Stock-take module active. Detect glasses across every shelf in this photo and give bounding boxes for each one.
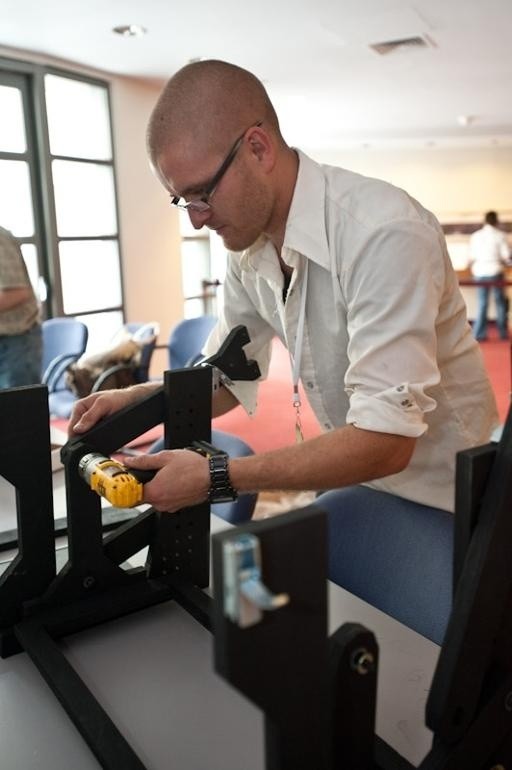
[170,123,263,211]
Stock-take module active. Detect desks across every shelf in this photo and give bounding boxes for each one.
[0,421,454,769]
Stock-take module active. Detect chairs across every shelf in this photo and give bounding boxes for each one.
[315,486,454,649]
[49,323,157,419]
[147,428,261,525]
[38,319,86,391]
[136,311,224,379]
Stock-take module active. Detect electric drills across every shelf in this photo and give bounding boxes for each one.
[77,440,228,509]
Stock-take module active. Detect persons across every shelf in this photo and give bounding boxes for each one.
[465,211,512,342]
[0,225,43,389]
[67,60,499,514]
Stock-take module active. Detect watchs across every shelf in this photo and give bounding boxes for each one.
[206,449,238,504]
